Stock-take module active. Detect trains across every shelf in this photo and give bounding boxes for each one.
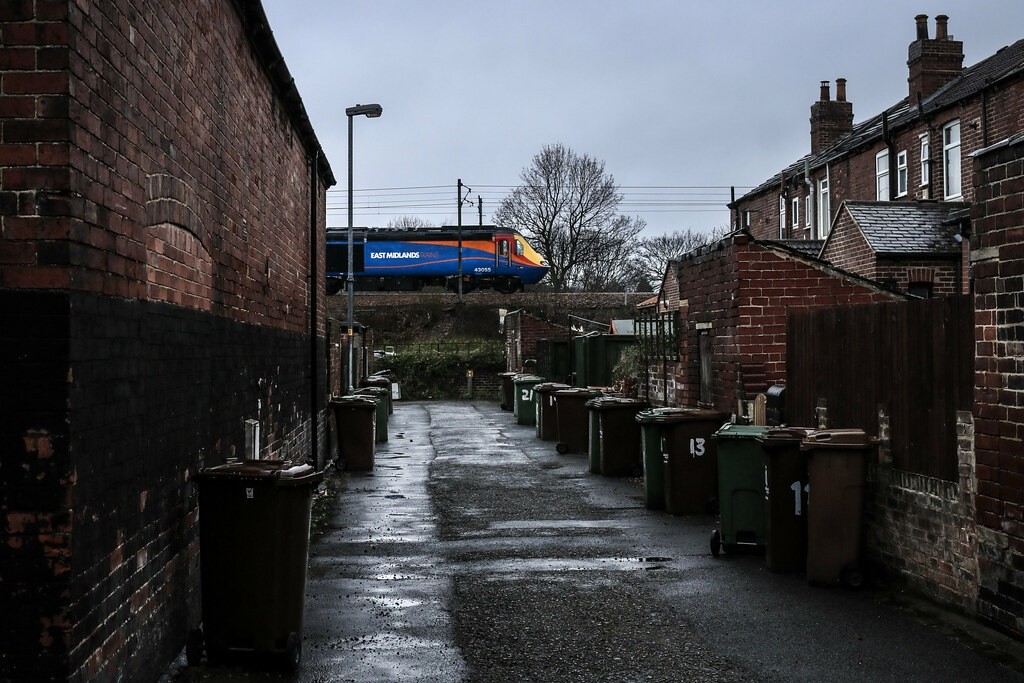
[325,224,551,297]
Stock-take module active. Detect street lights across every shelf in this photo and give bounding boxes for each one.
[346,102,383,393]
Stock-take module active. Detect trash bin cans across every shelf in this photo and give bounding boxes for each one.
[512,373,546,425]
[555,388,603,454]
[360,369,393,414]
[634,406,724,516]
[708,423,804,571]
[332,396,376,473]
[584,397,641,477]
[353,386,388,444]
[499,371,517,411]
[184,460,324,671]
[532,382,571,441]
[802,430,880,599]
